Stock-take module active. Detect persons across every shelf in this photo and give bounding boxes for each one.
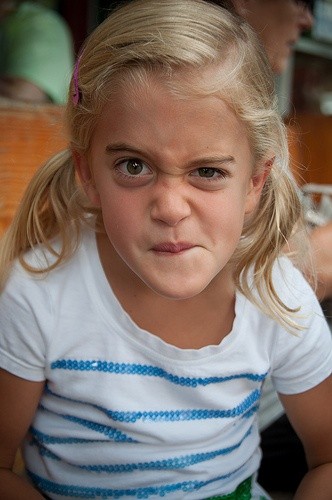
[2,0,76,106]
[2,0,332,498]
